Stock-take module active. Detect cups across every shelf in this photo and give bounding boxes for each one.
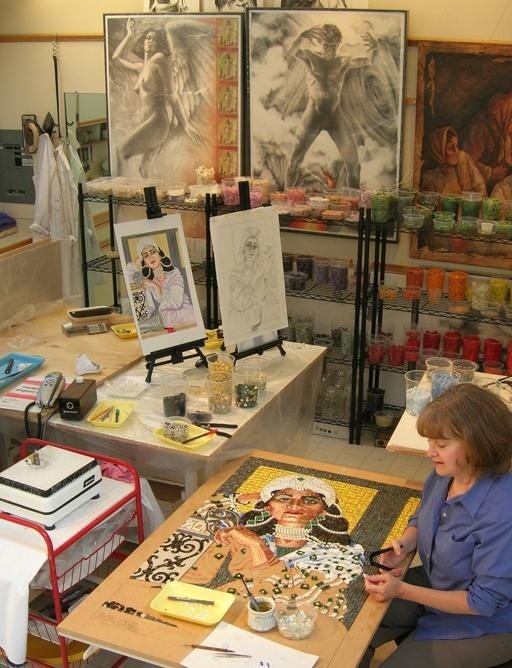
[246,596,279,633]
[111,306,121,323]
[272,603,318,640]
[159,350,269,421]
[87,172,511,238]
[281,255,512,427]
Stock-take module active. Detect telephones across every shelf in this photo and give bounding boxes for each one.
[34,371,65,408]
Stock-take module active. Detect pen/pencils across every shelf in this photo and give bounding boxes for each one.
[168,596,214,606]
[5,359,13,374]
[182,429,217,444]
[90,406,119,423]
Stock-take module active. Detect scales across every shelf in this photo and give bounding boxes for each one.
[0,444,103,530]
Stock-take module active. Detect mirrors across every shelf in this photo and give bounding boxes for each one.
[63,91,115,307]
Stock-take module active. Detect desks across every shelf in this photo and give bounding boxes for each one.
[0,302,143,455]
[386,364,512,456]
[44,325,329,547]
[57,444,425,667]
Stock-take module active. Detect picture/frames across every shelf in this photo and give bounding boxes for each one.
[103,9,244,189]
[245,7,407,244]
[409,37,512,276]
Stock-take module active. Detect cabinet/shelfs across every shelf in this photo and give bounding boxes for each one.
[78,184,222,329]
[356,204,512,448]
[0,434,148,668]
[211,196,386,447]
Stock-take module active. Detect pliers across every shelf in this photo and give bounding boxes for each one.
[192,421,238,438]
[364,545,403,571]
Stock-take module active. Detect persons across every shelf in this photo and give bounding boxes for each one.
[419,89,512,203]
[111,19,207,180]
[362,384,512,668]
[210,474,366,606]
[134,237,196,332]
[278,22,381,189]
[224,224,281,330]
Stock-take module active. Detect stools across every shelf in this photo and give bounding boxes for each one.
[391,631,512,667]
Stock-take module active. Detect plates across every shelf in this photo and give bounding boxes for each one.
[113,322,140,339]
[154,420,216,450]
[149,578,235,626]
[108,374,150,398]
[85,399,136,428]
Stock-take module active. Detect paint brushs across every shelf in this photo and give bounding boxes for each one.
[191,644,251,658]
